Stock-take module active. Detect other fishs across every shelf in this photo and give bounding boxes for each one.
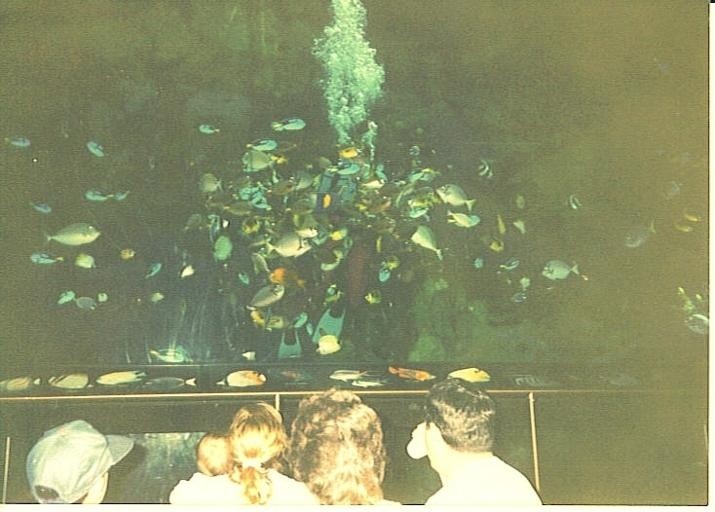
[145,116,483,365]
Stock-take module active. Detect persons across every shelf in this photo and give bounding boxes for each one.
[411,376,544,507]
[169,387,404,507]
[24,417,136,505]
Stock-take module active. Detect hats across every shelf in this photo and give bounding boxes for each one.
[26,420,134,504]
